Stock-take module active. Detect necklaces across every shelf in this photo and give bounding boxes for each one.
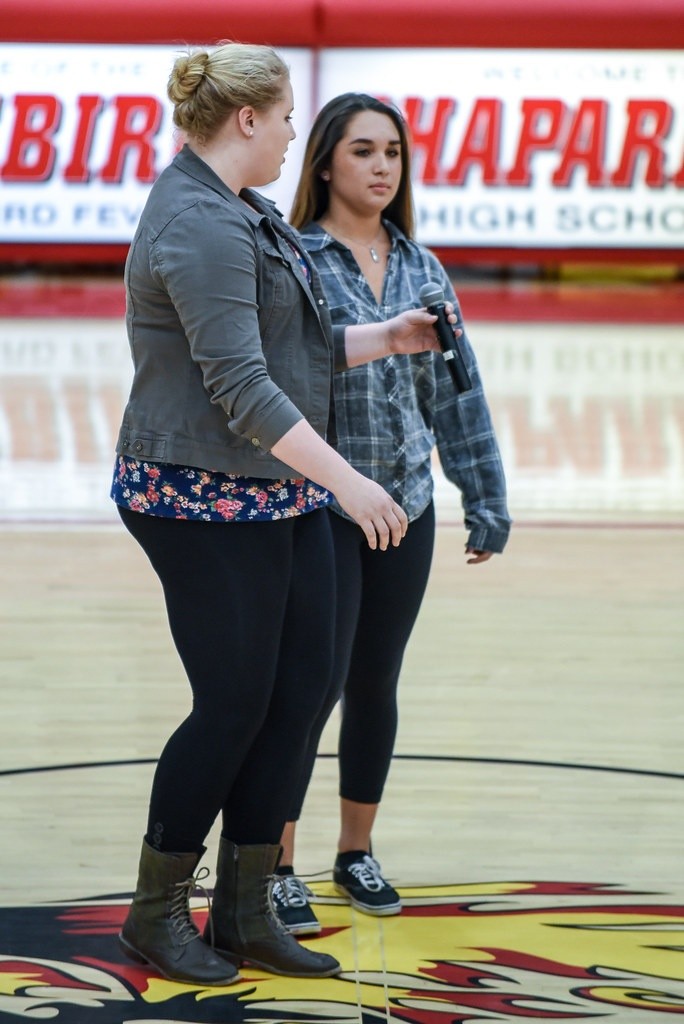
[320,215,382,262]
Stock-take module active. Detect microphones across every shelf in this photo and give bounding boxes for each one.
[418,281,474,394]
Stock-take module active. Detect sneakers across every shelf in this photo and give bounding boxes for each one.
[332,851,402,917]
[270,871,322,936]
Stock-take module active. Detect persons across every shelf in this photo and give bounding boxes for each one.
[268,91,512,932]
[110,43,463,981]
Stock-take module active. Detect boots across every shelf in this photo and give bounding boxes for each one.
[116,833,243,987]
[202,834,343,979]
